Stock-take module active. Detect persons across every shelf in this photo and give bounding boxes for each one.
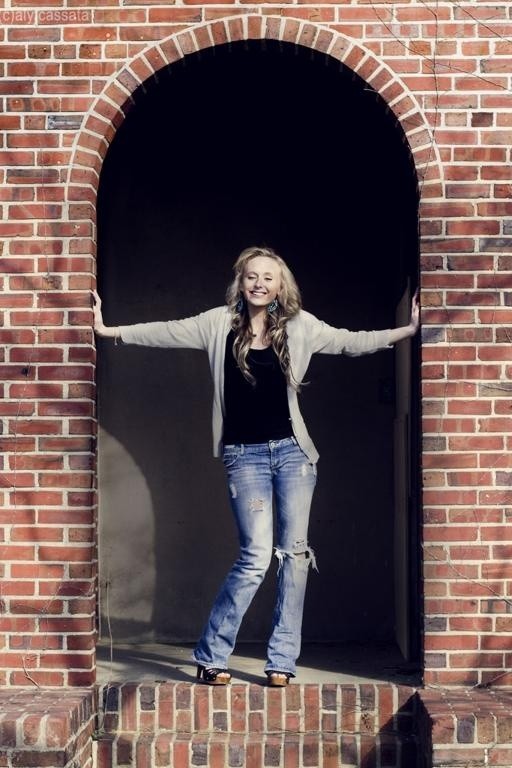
[89,245,421,685]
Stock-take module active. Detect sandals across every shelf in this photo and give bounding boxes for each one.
[196,662,233,684]
[266,670,291,687]
